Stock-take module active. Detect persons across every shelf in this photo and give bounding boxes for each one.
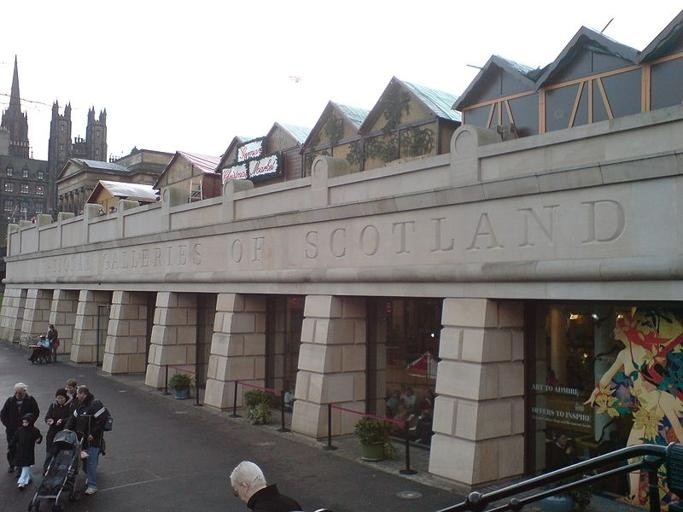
[28,324,58,362]
[0,378,112,495]
[583,312,683,504]
[283,387,293,413]
[545,428,588,501]
[387,387,435,438]
[230,460,300,512]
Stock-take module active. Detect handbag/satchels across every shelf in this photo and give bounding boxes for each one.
[103,414,114,432]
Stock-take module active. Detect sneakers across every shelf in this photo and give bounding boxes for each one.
[7,464,34,490]
[84,475,98,496]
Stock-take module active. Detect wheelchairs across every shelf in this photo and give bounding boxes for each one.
[31,340,52,365]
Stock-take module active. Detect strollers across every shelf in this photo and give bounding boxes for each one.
[27,415,93,512]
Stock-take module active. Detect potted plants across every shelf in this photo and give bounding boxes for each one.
[354,412,393,461]
[169,373,193,400]
